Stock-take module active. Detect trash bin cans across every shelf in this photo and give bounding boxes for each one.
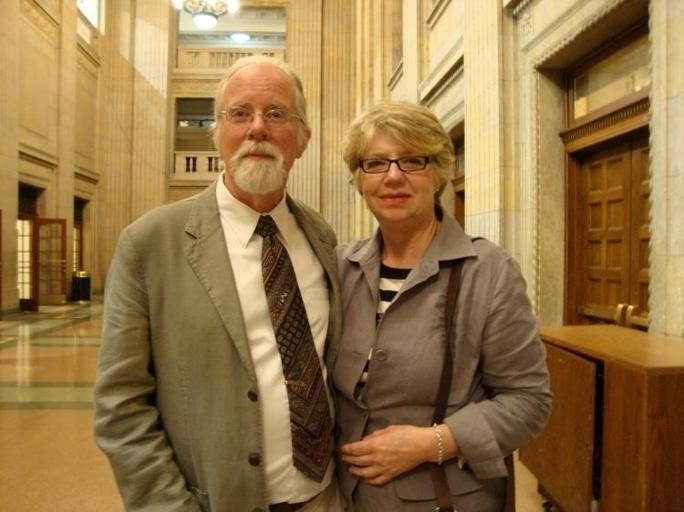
[72,271,91,304]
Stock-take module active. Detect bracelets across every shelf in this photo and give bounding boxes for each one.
[433,422,443,464]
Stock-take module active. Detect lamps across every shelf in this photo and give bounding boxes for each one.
[174,0,238,28]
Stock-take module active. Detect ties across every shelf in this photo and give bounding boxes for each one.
[254,214,338,484]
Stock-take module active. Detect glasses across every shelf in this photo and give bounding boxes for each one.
[218,106,304,123]
[360,155,437,174]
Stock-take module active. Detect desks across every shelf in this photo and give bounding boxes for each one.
[518,324,682,511]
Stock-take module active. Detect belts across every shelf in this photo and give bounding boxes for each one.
[269,501,309,512]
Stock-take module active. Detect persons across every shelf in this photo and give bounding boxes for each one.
[91,55,345,512]
[334,99,554,511]
